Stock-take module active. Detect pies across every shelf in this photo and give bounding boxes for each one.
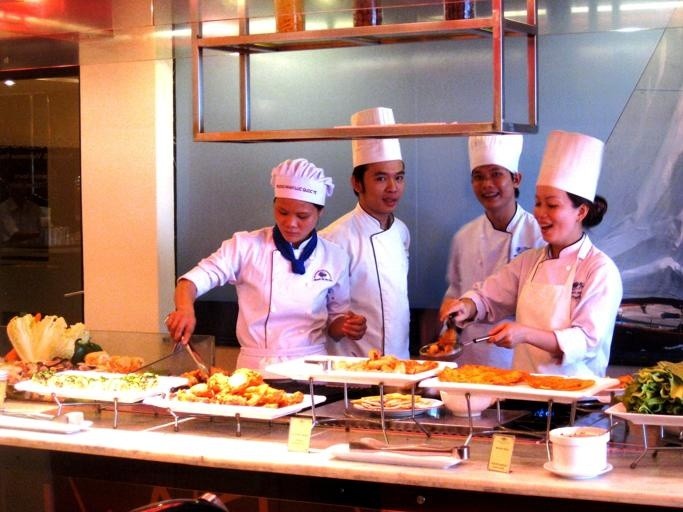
[437,364,595,391]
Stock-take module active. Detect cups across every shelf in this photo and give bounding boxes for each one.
[274,2,305,32]
[350,0,383,26]
[443,2,474,22]
[548,425,609,469]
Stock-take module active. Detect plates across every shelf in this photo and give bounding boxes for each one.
[544,460,613,478]
[145,384,325,423]
[606,401,680,425]
[265,355,457,388]
[352,397,443,416]
[418,370,617,405]
[56,367,192,407]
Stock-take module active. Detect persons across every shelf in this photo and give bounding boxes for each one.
[163,158,367,382]
[434,164,549,370]
[438,184,623,432]
[316,160,413,362]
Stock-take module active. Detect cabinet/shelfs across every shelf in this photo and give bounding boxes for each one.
[187,1,541,145]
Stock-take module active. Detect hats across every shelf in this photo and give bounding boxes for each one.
[270,158,335,206]
[467,135,524,176]
[350,106,403,169]
[535,129,606,206]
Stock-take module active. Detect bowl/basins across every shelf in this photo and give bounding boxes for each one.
[440,389,493,418]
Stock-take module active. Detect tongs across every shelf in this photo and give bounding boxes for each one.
[162,316,212,378]
